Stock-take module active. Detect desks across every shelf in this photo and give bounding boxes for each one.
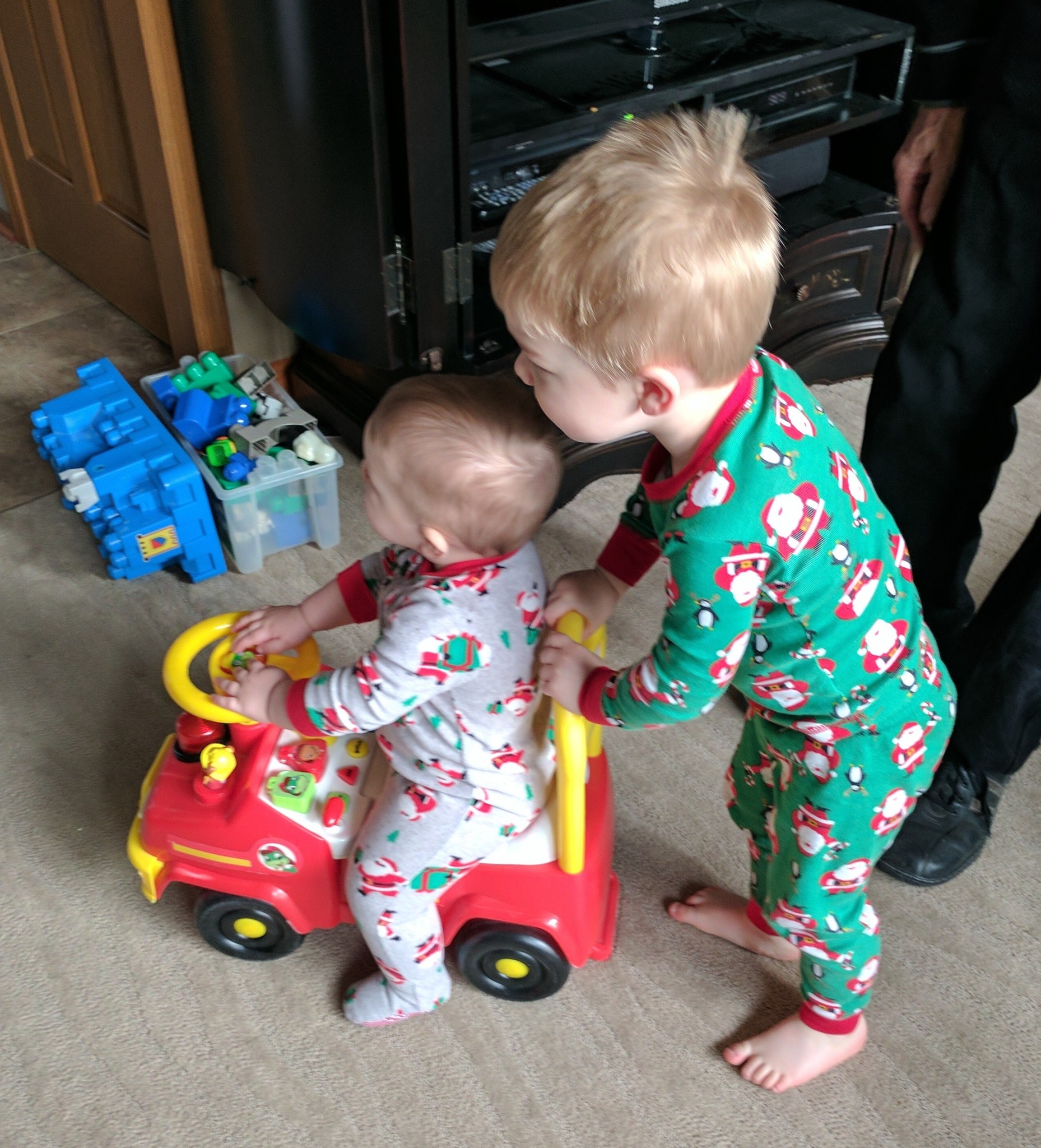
[159,0,979,527]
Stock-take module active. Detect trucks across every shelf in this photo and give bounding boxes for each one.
[127,612,620,1003]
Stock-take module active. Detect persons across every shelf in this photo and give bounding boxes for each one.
[858,0,1041,890]
[207,372,561,1027]
[490,108,957,1091]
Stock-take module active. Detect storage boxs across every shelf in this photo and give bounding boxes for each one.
[139,353,345,576]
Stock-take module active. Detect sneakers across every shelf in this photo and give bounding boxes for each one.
[879,761,1013,888]
